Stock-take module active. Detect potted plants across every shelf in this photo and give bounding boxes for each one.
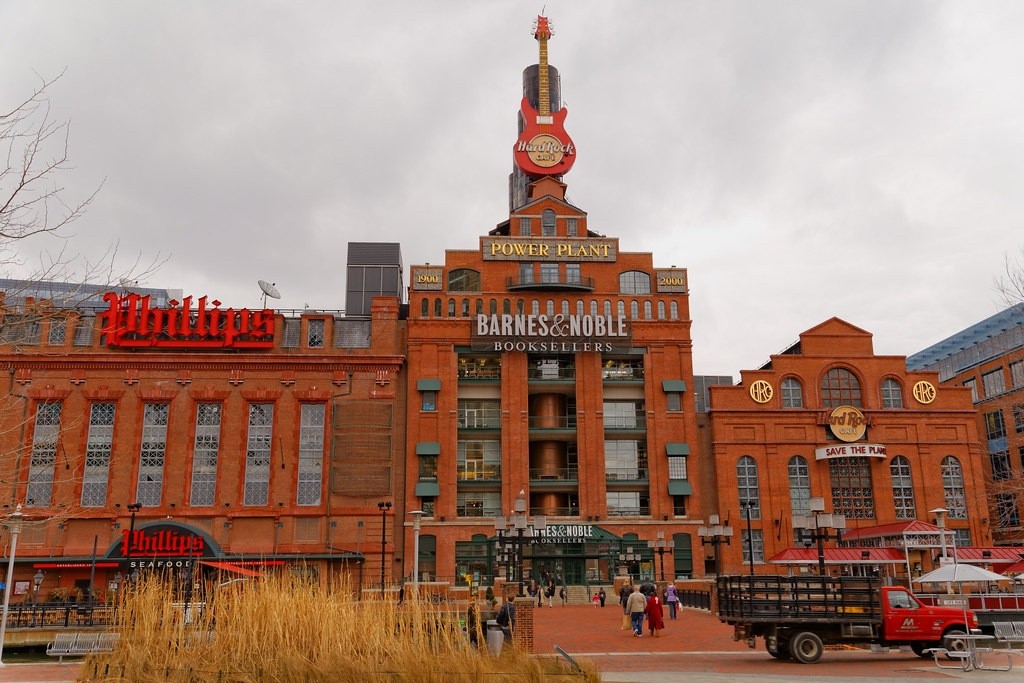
[486,587,498,610]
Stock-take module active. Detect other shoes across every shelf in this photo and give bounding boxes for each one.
[657,636,659,638]
[550,606,552,609]
[617,603,620,605]
[633,629,638,636]
[538,603,540,606]
[650,632,654,635]
[637,634,642,637]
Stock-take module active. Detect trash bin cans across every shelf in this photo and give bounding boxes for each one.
[870,645,889,653]
[486,620,504,658]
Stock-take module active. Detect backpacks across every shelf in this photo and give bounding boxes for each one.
[496,604,511,626]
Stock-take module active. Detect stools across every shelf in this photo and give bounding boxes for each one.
[927,647,1012,671]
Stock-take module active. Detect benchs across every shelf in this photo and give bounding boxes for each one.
[45,634,120,663]
[992,621,1024,643]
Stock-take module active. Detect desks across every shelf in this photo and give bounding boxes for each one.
[945,634,994,670]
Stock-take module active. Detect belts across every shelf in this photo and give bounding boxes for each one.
[531,591,534,592]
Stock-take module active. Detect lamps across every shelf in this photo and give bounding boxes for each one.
[983,550,991,559]
[805,542,812,548]
[982,519,987,525]
[597,515,600,521]
[441,517,444,522]
[862,551,870,559]
[76,607,86,614]
[725,520,729,525]
[589,516,593,521]
[775,520,779,525]
[933,519,937,524]
[665,516,668,520]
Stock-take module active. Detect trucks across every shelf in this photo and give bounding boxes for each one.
[716,573,979,664]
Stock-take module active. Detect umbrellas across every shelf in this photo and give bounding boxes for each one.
[912,564,1013,635]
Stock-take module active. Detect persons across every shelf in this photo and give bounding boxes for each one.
[593,587,606,607]
[398,586,404,606]
[618,579,633,630]
[500,595,516,639]
[640,577,657,620]
[1005,587,1009,593]
[528,580,537,597]
[626,585,647,637]
[468,601,478,645]
[537,573,567,608]
[665,581,677,620]
[644,592,663,638]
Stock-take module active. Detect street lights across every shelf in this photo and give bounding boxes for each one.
[619,547,641,586]
[647,531,674,581]
[697,514,733,581]
[495,500,547,596]
[33,570,44,607]
[792,497,846,588]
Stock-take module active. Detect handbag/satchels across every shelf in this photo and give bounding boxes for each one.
[620,612,632,630]
[676,600,683,612]
[544,591,550,598]
